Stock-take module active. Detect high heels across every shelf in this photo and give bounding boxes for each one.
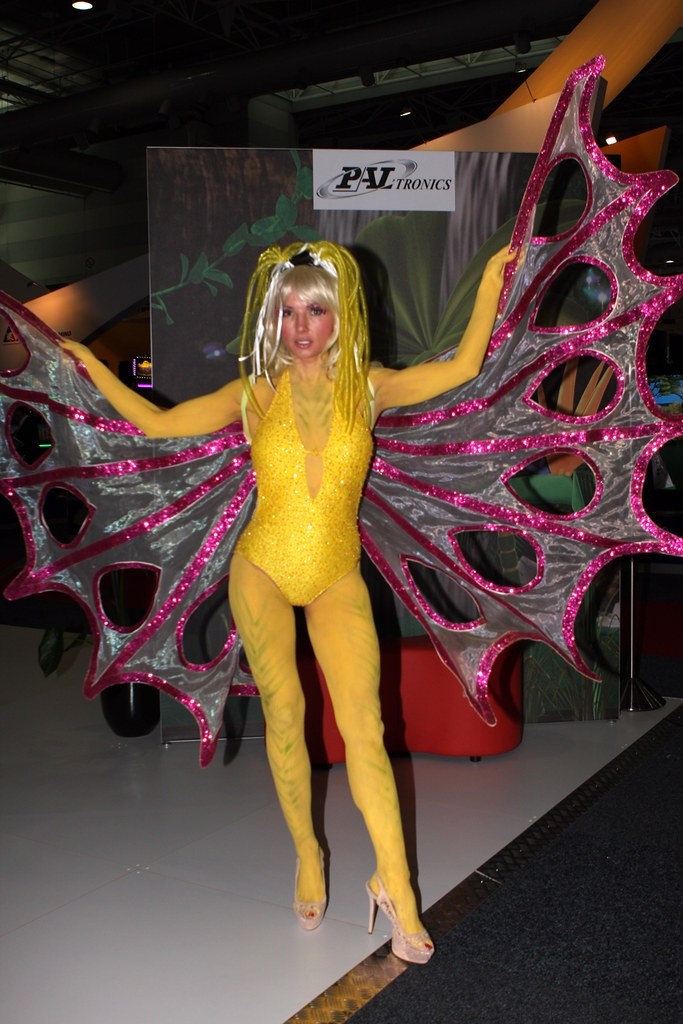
[294,847,326,930]
[365,872,435,963]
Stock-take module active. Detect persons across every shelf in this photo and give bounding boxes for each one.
[55,242,521,965]
